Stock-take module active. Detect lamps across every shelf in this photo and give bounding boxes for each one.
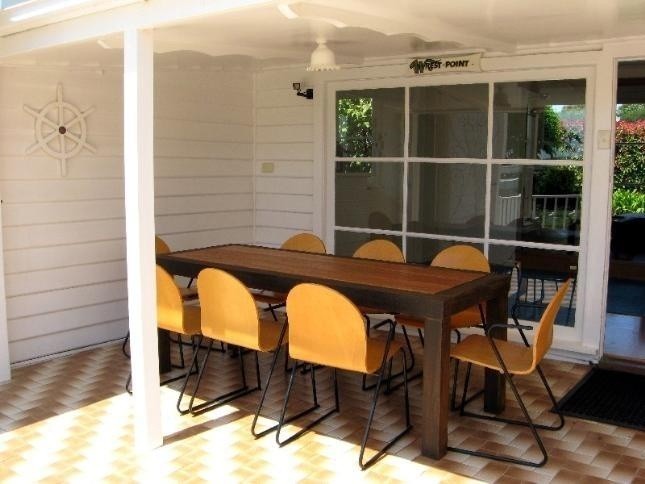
[303,37,342,75]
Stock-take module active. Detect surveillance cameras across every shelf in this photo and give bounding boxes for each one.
[292,80,302,90]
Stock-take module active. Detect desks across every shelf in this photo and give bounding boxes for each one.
[156,243,508,462]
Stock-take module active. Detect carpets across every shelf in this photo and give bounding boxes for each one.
[545,355,644,436]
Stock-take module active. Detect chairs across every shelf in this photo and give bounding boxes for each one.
[125,263,246,412]
[247,234,325,373]
[123,234,226,369]
[277,282,411,469]
[187,268,321,438]
[336,202,575,326]
[445,279,570,469]
